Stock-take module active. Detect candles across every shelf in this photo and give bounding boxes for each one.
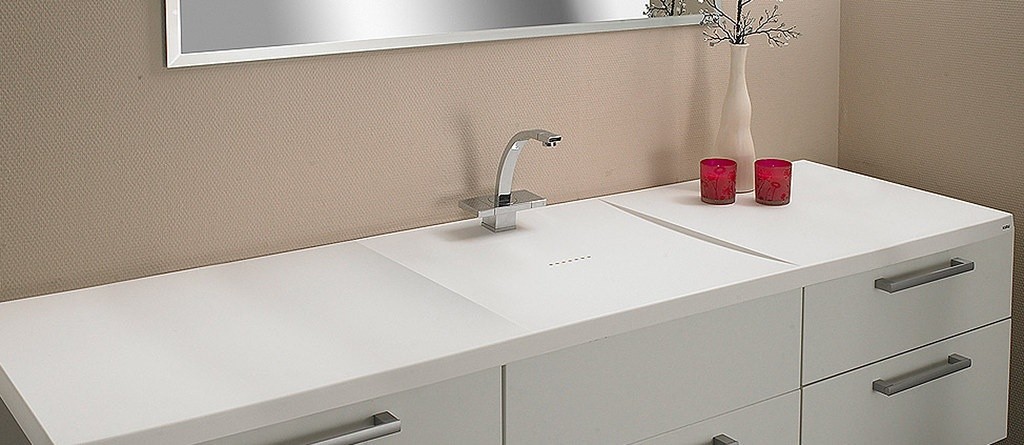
[700,158,737,205]
[755,159,792,205]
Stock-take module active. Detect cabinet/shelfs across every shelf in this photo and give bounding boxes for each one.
[96,215,1015,445]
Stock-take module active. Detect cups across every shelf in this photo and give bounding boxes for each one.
[700,158,737,205]
[754,158,792,206]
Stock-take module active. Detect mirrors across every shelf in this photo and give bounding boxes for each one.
[166,0,722,69]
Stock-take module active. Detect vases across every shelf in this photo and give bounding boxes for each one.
[714,42,757,192]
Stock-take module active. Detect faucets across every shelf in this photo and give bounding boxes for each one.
[458,129,563,233]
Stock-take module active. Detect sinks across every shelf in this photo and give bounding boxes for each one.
[358,195,797,328]
